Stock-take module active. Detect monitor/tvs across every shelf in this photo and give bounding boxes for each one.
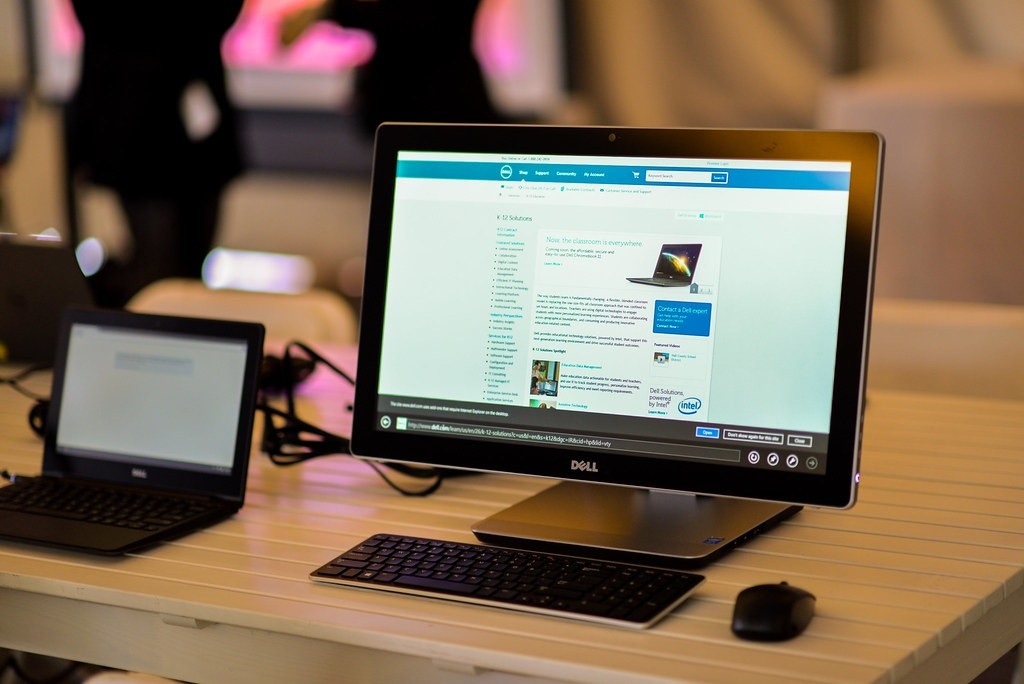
[350,122,886,569]
[544,380,557,392]
[626,244,702,287]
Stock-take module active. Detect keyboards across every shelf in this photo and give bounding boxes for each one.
[310,533,705,632]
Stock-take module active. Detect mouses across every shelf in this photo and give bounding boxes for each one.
[730,580,816,643]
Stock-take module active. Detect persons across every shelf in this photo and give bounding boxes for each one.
[269,1,501,148]
[531,360,552,385]
[57,1,249,289]
[531,377,539,394]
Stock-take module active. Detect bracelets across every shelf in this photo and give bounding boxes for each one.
[547,380,549,382]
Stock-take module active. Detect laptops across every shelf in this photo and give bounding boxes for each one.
[0,310,266,556]
[0,237,146,364]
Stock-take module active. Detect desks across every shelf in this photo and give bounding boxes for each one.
[0,365,1024,684]
